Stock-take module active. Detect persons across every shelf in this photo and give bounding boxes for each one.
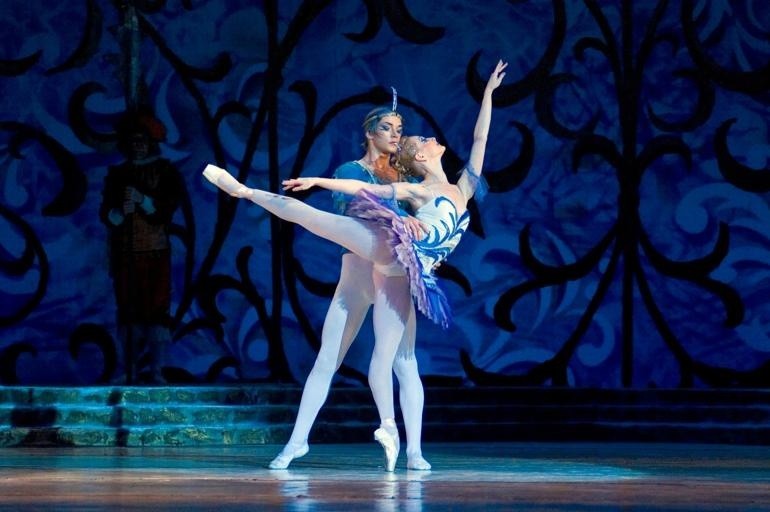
[266,106,434,470]
[202,59,509,471]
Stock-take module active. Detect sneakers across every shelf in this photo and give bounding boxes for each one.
[268,442,309,469]
[375,428,398,471]
[407,455,431,469]
[203,163,246,196]
